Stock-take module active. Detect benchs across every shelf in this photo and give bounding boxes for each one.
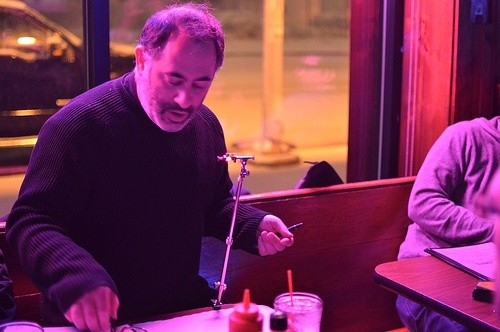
[0,110,419,332]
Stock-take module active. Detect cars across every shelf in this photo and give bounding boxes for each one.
[0,0,146,175]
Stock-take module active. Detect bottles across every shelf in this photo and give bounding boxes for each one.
[229,289,264,332]
[270,312,287,332]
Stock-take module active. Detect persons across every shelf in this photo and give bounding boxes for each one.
[5,2,294,332]
[0,249,16,326]
[396,116,500,332]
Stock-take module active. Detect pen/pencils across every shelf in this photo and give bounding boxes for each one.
[275,222,304,238]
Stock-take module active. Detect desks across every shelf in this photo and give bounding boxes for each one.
[109,303,274,332]
[374,255,500,332]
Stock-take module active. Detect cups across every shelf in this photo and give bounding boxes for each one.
[274,292,323,332]
